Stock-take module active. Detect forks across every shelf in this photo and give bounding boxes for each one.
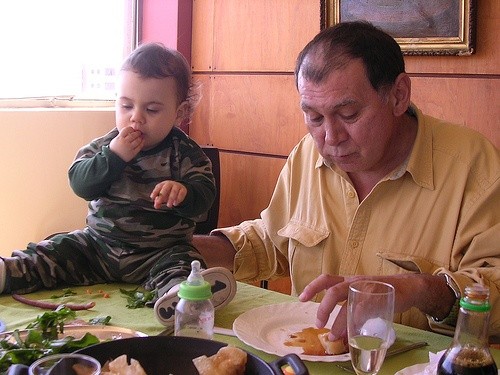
[335,341,428,375]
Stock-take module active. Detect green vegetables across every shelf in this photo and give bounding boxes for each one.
[0,284,158,371]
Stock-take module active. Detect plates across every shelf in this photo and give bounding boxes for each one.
[0,325,149,344]
[233,301,351,362]
[394,362,428,375]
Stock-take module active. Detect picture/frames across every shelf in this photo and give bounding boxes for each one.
[320,0,475,56]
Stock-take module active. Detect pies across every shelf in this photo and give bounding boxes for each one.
[318,330,349,355]
[192,345,247,375]
[71,355,148,375]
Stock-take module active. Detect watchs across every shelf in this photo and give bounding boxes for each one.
[432,274,461,325]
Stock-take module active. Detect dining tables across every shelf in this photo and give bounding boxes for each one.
[0,280,500,375]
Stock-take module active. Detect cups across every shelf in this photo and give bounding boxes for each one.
[28,354,101,375]
[346,280,395,375]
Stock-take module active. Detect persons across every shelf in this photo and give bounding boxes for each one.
[1,43,237,327]
[192,18,500,345]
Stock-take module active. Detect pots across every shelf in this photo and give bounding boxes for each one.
[6,336,310,375]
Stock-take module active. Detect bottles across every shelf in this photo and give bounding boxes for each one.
[437,283,498,375]
[174,261,214,339]
